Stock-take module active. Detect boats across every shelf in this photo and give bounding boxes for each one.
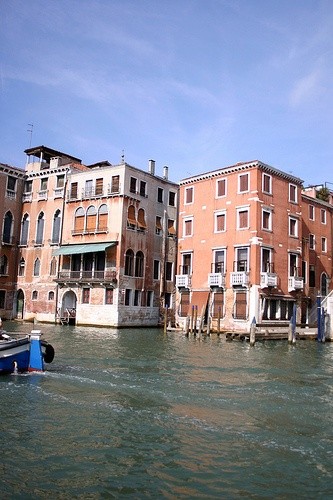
[0,330,54,376]
